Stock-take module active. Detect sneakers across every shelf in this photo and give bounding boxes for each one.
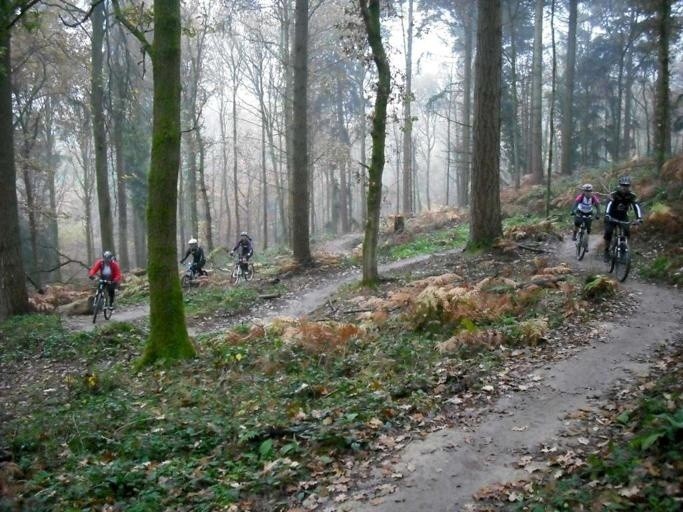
[604,257,608,262]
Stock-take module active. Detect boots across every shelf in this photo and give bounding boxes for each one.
[572,231,577,240]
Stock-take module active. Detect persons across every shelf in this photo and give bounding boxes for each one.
[179,238,206,276]
[570,184,600,242]
[229,230,254,267]
[88,251,122,310]
[603,176,644,264]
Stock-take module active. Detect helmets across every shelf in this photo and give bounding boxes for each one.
[619,176,631,185]
[240,231,248,236]
[582,184,593,190]
[103,251,112,258]
[188,239,198,244]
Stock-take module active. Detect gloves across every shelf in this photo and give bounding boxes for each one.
[604,215,611,222]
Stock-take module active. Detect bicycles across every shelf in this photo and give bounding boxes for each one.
[605,218,639,283]
[572,214,595,261]
[92,278,112,324]
[229,255,254,288]
[180,262,208,304]
